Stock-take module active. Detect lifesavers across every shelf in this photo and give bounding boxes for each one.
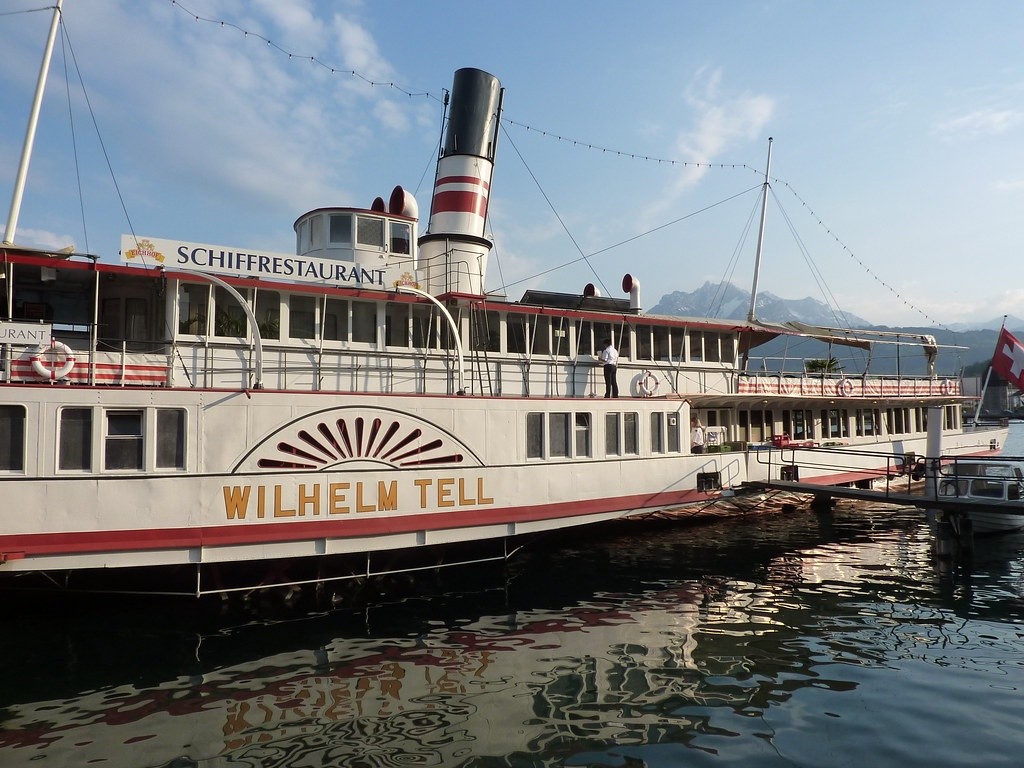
[841,378,853,397]
[31,342,76,380]
[640,372,660,396]
[945,380,954,395]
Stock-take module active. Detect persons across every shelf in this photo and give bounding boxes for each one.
[598,338,619,398]
[690,416,704,454]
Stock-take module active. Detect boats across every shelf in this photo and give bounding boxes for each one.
[932,418,1023,537]
[2,0,1011,640]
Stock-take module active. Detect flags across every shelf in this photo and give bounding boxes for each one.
[990,325,1024,391]
